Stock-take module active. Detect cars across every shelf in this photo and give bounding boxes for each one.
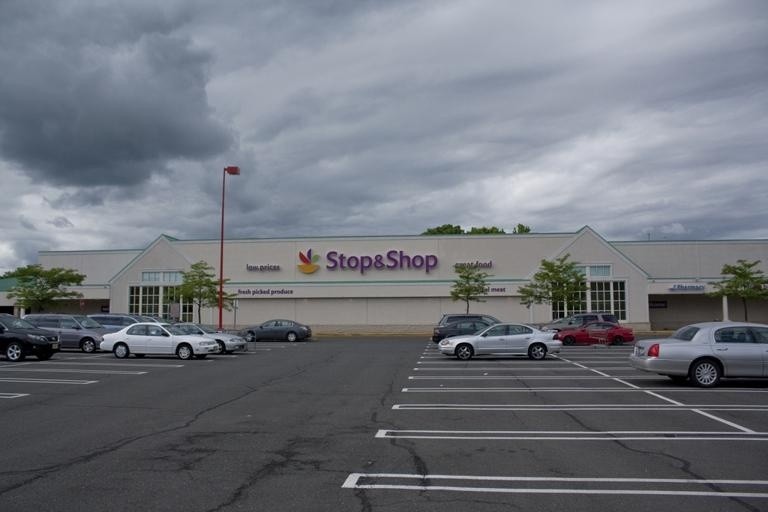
[0,311,247,360]
[241,319,312,342]
[627,321,768,386]
[431,312,634,360]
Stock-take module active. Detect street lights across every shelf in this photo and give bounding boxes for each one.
[218,165,240,333]
